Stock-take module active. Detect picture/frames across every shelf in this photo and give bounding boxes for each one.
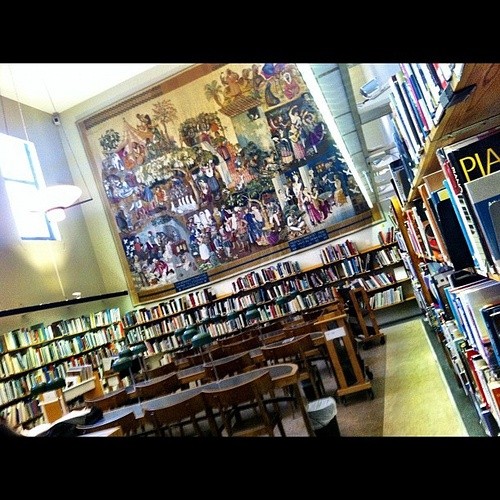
[74,63,387,309]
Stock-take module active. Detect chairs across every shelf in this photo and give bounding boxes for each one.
[75,316,323,438]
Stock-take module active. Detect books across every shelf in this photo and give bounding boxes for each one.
[387,63,500,436]
[0,308,126,430]
[126,226,405,367]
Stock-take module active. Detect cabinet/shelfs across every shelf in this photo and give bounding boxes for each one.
[397,62,500,439]
[126,242,415,367]
[0,307,129,436]
